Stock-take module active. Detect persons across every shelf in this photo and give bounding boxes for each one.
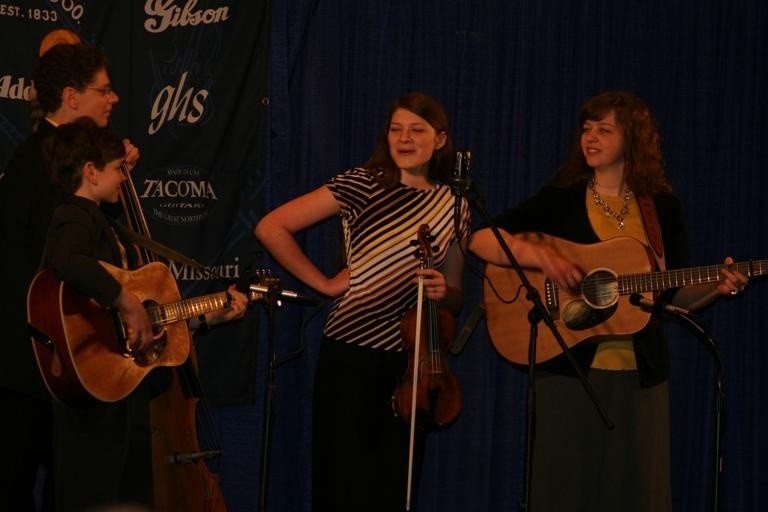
[0,40,147,512]
[463,89,752,512]
[251,91,472,511]
[35,114,251,512]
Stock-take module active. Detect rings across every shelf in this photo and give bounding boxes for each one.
[728,288,738,298]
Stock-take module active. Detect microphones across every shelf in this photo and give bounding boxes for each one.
[235,277,320,306]
[629,293,689,315]
[451,151,471,196]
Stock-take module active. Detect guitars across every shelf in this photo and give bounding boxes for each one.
[27,257,280,407]
[483,231,766,366]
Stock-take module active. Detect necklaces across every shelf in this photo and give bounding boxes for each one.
[585,181,636,231]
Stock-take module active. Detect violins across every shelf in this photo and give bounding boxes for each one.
[393,225,463,427]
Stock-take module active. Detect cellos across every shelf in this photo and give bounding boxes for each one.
[118,161,227,510]
